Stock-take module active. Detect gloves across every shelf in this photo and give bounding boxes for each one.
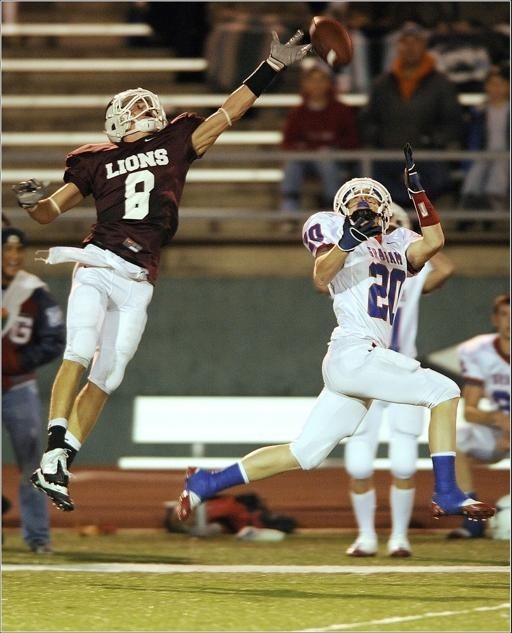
[267,28,312,72]
[11,179,45,209]
[401,143,426,200]
[337,215,381,252]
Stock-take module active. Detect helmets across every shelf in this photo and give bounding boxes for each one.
[333,177,393,235]
[101,87,169,143]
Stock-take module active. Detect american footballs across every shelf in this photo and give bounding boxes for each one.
[309,15,354,68]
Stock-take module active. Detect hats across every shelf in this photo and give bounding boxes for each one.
[2,228,28,248]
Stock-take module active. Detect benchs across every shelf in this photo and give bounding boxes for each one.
[118,393,511,535]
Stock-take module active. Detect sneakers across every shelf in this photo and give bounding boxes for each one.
[40,448,72,485]
[387,533,411,557]
[346,535,378,556]
[29,468,73,512]
[432,496,496,522]
[448,526,482,538]
[176,467,204,522]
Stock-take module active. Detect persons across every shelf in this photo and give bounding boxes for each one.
[459,69,508,205]
[127,0,511,86]
[342,199,456,558]
[2,230,67,560]
[285,62,359,219]
[444,292,511,539]
[362,28,468,203]
[13,28,313,513]
[178,140,494,520]
